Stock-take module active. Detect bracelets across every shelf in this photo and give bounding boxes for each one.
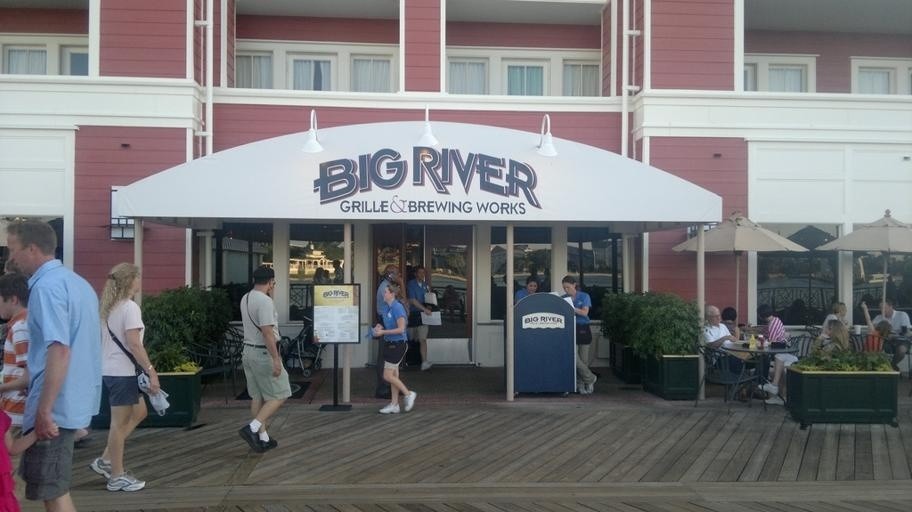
[145,365,152,373]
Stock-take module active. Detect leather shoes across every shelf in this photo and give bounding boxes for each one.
[239,425,263,453]
[258,436,278,452]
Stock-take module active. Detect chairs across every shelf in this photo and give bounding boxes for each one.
[790,335,815,360]
[805,324,821,336]
[851,334,895,359]
[187,342,245,405]
[694,345,767,414]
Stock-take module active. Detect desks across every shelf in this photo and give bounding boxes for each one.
[723,344,799,411]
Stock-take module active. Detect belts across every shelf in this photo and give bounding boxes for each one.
[243,343,267,349]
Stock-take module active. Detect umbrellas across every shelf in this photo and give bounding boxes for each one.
[759,225,868,321]
[812,209,912,320]
[670,209,811,341]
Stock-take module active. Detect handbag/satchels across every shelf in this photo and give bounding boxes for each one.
[138,372,170,417]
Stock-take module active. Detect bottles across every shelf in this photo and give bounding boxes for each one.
[309,237,313,251]
[749,335,757,349]
[756,335,764,350]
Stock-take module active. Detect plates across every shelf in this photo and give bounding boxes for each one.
[852,326,869,329]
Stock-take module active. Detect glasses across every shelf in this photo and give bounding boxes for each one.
[710,314,720,318]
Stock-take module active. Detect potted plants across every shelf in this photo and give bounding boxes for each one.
[784,349,901,429]
[599,287,708,401]
[92,318,203,428]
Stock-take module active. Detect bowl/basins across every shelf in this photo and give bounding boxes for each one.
[733,342,744,346]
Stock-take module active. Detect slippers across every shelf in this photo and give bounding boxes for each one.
[73,431,93,447]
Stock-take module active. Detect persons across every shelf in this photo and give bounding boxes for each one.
[553,275,598,397]
[0,218,101,511]
[406,267,436,371]
[372,286,417,415]
[698,298,912,406]
[513,278,542,310]
[238,265,292,452]
[312,256,344,285]
[376,265,406,399]
[87,263,159,492]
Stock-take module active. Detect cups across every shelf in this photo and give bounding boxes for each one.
[854,325,861,335]
[900,322,907,334]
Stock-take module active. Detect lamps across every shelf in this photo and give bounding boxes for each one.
[536,113,556,156]
[417,108,437,147]
[302,108,323,152]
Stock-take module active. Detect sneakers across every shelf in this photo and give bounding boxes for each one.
[576,386,587,395]
[751,383,768,400]
[764,394,786,406]
[379,403,400,414]
[375,391,392,399]
[585,373,598,394]
[89,457,112,482]
[891,361,900,372]
[757,382,779,396]
[404,390,417,412]
[106,472,146,492]
[738,386,747,402]
[421,361,433,372]
